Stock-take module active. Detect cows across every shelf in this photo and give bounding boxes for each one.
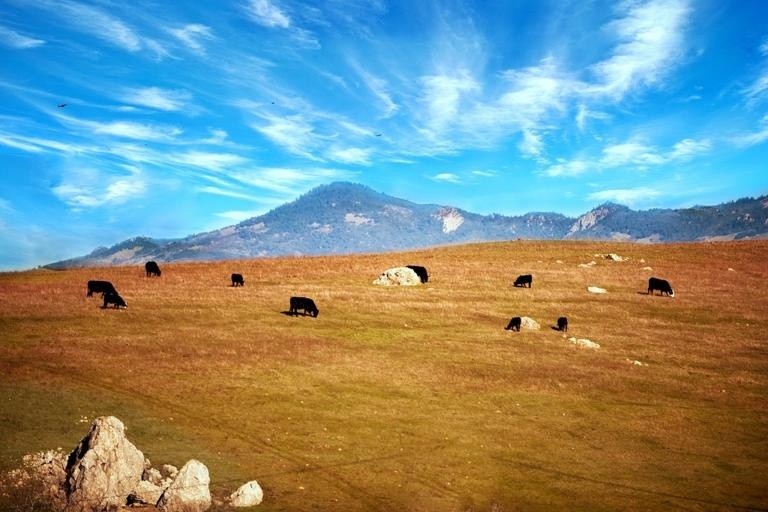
[146,262,161,277]
[648,277,674,296]
[87,280,127,310]
[407,266,430,284]
[232,274,245,287]
[290,297,319,318]
[507,317,521,331]
[558,317,567,332]
[514,275,532,288]
